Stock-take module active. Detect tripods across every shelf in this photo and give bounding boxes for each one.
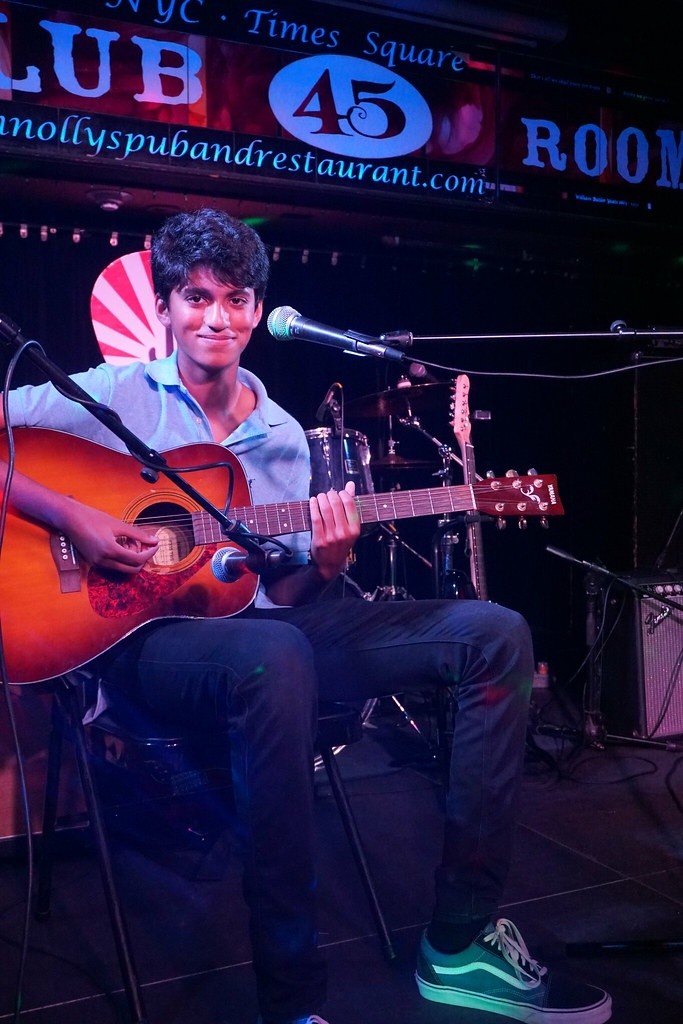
[357,475,417,727]
[545,544,683,790]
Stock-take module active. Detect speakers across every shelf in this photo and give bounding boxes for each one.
[603,573,683,744]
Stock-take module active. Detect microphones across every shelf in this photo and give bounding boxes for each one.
[212,546,294,583]
[316,384,338,423]
[410,363,439,384]
[267,305,405,362]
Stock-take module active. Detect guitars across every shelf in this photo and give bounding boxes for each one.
[0,426,565,684]
[448,372,490,601]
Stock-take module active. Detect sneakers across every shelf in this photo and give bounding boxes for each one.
[256,1012,331,1024]
[417,913,615,1024]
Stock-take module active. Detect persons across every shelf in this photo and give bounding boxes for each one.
[0,208,612,1024]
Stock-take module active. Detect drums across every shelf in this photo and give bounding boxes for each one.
[304,426,377,540]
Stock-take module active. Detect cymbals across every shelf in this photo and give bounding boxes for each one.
[371,451,445,472]
[340,381,455,419]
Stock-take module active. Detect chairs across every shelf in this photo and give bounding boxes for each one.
[32,667,400,1024]
